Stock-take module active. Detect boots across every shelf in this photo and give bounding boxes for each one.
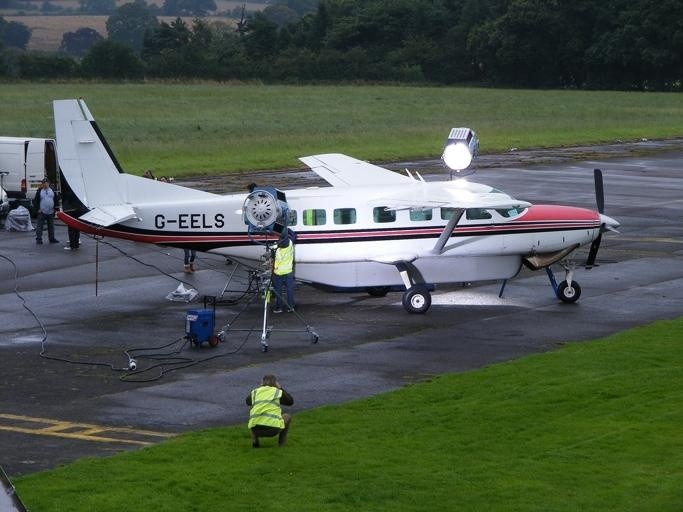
[190,262,196,272]
[184,264,192,274]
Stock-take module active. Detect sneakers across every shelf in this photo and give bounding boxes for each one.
[252,441,260,447]
[278,438,287,446]
[78,238,82,244]
[288,307,294,312]
[64,246,72,251]
[36,238,43,244]
[273,309,282,313]
[49,239,59,243]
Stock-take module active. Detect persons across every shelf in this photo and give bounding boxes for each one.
[182,247,195,274]
[32,177,58,245]
[245,373,294,449]
[269,227,297,313]
[61,223,79,252]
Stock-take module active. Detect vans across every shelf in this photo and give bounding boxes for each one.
[0,134,59,215]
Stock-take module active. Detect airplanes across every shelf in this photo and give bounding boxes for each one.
[50,97,622,315]
